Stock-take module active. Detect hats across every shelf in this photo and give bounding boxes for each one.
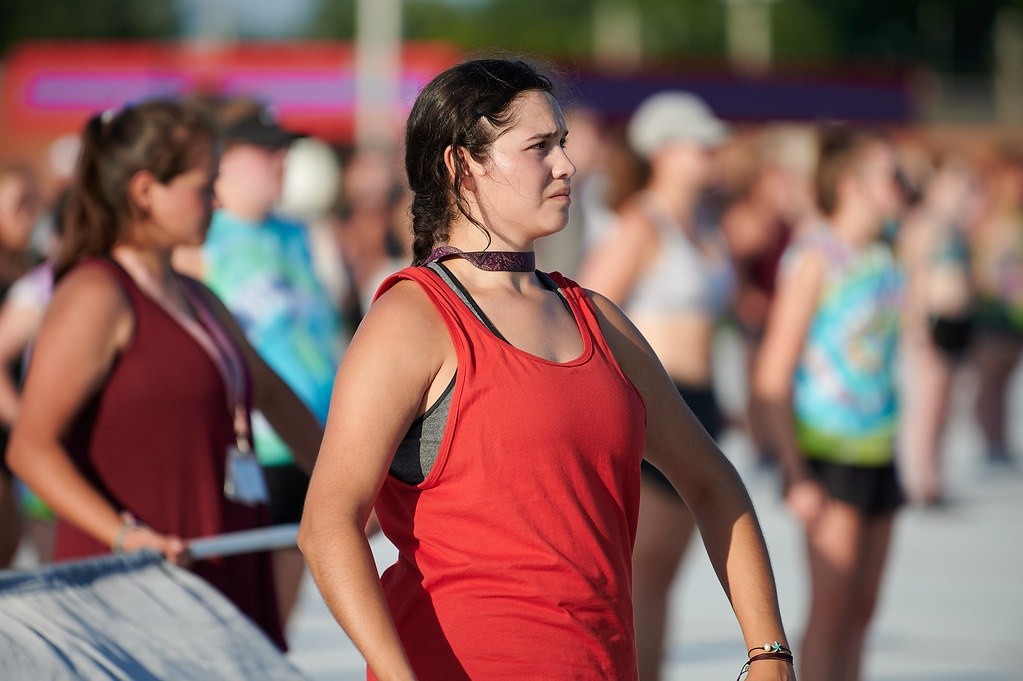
[218,112,309,157]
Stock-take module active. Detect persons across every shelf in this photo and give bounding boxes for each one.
[0,89,413,655]
[299,59,796,681]
[536,88,1023,681]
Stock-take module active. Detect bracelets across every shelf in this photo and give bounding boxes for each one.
[736,641,794,681]
[110,523,143,555]
